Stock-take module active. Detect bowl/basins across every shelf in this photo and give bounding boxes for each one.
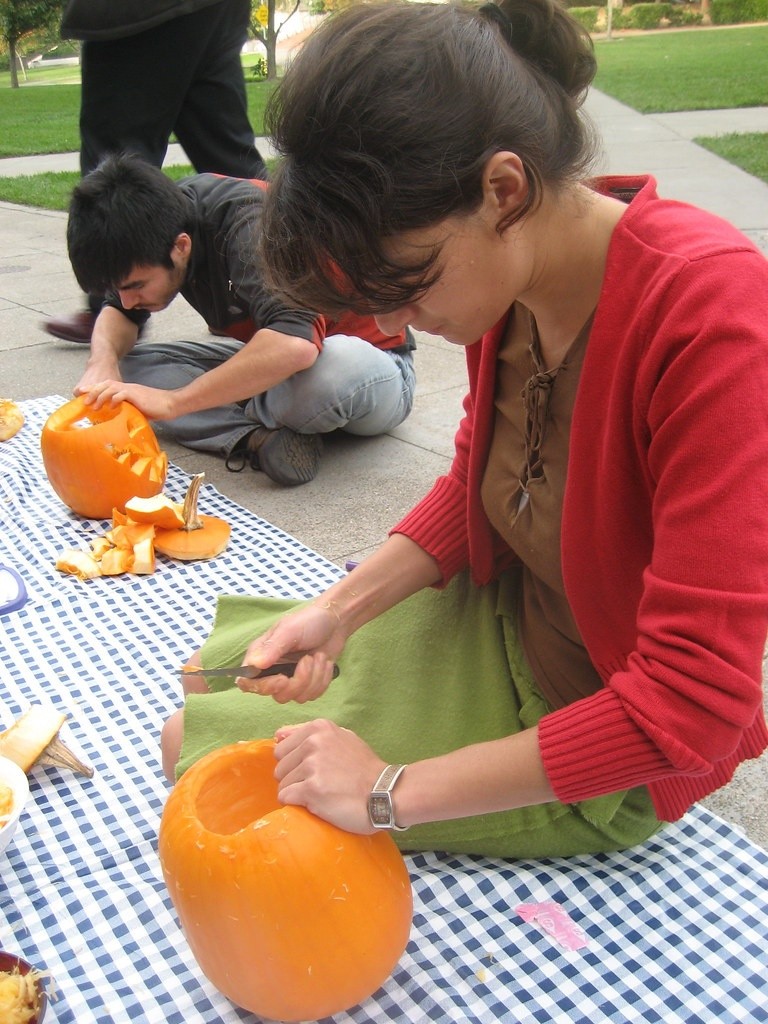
[0,951,48,1024]
[0,754,29,857]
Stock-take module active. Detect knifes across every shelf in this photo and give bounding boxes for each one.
[171,661,343,681]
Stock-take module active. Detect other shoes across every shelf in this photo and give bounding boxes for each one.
[226,421,325,485]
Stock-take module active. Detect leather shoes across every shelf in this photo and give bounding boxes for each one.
[40,309,141,343]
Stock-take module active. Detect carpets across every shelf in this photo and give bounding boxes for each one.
[0,391,768,1023]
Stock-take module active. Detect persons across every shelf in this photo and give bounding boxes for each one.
[163,0,768,855]
[42,0,274,345]
[65,156,419,486]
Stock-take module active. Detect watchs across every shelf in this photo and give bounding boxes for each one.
[367,764,410,834]
